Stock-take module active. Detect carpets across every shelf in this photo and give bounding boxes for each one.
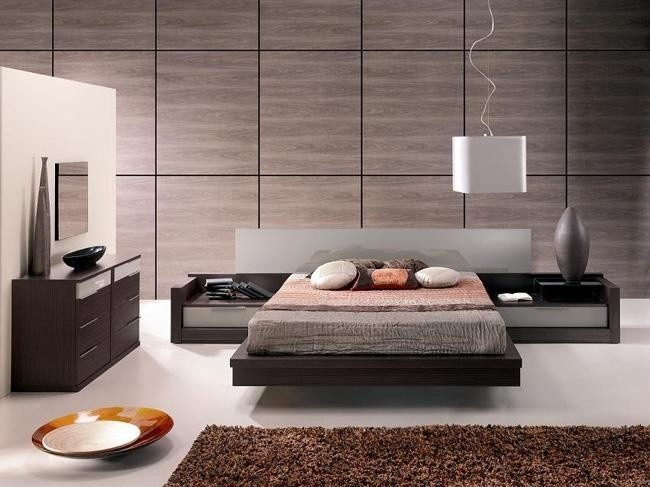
[163,425,650,487]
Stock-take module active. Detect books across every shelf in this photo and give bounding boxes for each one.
[203,275,274,299]
[497,292,534,302]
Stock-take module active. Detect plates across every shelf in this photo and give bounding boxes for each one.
[30,406,175,462]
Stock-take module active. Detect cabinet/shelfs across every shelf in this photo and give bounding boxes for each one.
[12,254,141,392]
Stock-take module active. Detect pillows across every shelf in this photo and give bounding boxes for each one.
[305,258,461,290]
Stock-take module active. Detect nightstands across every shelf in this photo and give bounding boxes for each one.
[476,273,620,343]
[171,273,292,343]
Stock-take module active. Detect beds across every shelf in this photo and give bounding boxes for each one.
[231,272,522,386]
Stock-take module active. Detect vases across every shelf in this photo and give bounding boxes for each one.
[33,157,50,275]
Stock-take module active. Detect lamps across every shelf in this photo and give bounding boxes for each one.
[452,0,527,194]
